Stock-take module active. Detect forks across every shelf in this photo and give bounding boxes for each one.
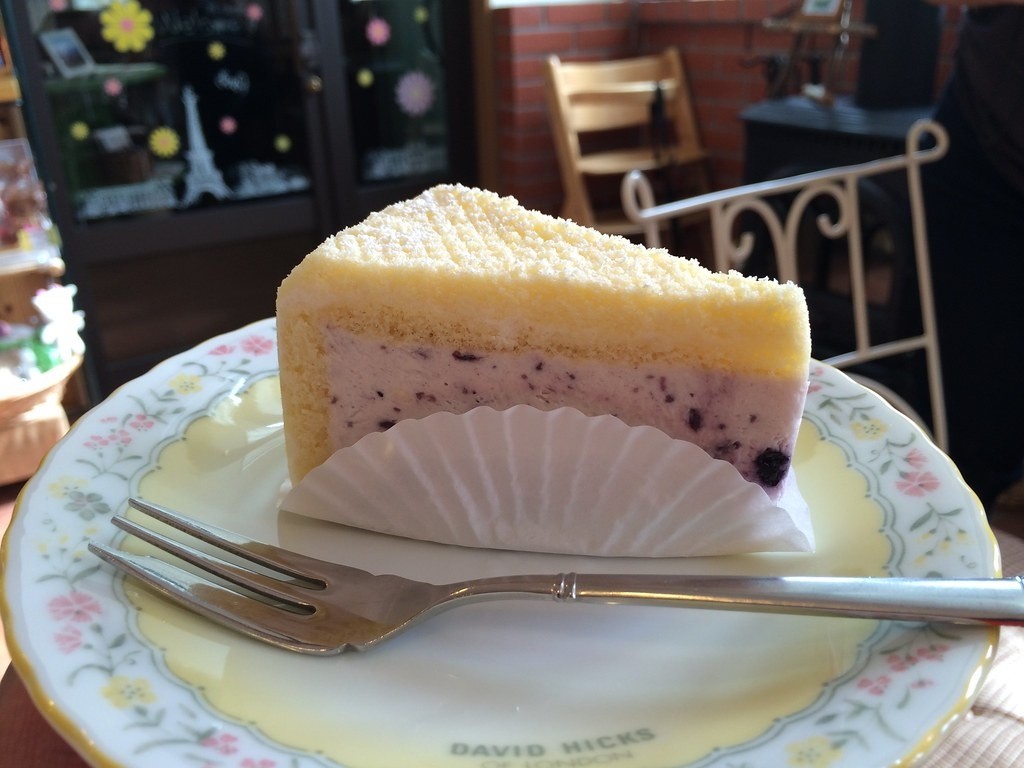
[87,498,1023,656]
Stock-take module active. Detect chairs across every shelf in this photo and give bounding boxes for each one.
[623,118,950,452]
[545,43,716,235]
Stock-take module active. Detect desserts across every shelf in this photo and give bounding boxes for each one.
[274,181,817,556]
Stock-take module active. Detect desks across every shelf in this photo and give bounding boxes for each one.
[739,90,935,328]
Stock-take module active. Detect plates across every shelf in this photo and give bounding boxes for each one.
[0,315,1001,768]
